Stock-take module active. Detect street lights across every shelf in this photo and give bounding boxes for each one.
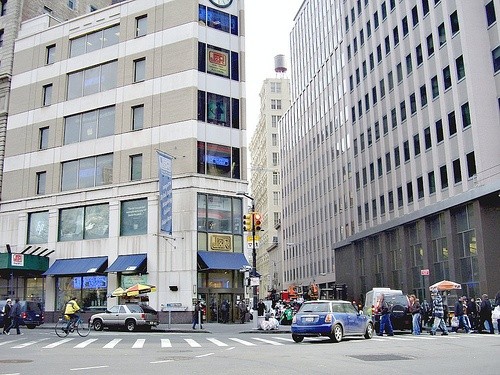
[236,191,261,330]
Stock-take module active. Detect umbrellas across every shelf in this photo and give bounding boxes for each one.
[124,283,157,296]
[429,280,461,291]
[106,287,128,297]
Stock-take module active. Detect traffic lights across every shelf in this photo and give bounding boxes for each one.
[254,213,262,233]
[243,214,252,231]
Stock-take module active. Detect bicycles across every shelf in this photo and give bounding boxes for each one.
[54,311,91,339]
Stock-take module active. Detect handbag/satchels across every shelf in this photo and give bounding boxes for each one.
[451,317,460,327]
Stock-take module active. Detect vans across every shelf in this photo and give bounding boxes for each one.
[363,288,403,323]
[0,299,45,329]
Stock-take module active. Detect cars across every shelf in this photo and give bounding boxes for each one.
[291,300,375,343]
[88,303,160,331]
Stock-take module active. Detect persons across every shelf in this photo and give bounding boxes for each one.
[209,102,223,120]
[375,293,394,336]
[406,292,449,335]
[192,301,203,329]
[220,299,229,323]
[258,299,266,316]
[451,292,500,335]
[275,298,303,324]
[1,298,13,334]
[4,299,24,335]
[239,299,248,323]
[64,297,82,331]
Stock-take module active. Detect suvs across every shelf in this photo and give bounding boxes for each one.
[375,294,413,336]
